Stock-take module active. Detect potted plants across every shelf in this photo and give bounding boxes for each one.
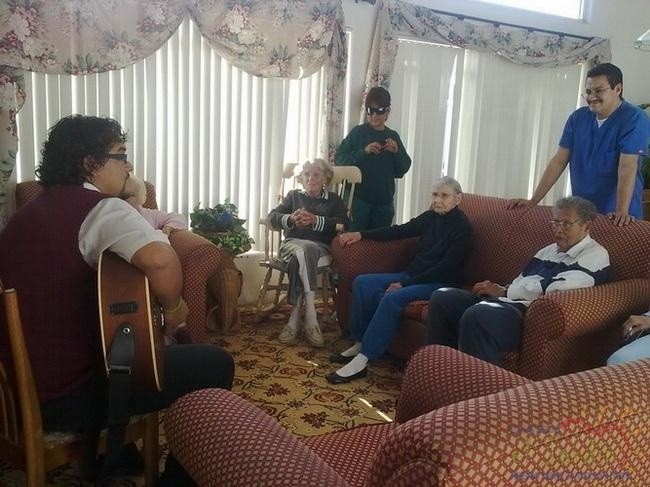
[187,200,257,335]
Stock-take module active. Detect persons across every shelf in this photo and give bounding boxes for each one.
[324,175,472,383]
[334,87,411,231]
[425,194,610,364]
[606,309,650,366]
[265,158,351,346]
[504,63,650,227]
[0,115,235,487]
[122,176,187,237]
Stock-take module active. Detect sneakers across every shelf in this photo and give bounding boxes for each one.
[115,458,144,471]
[301,325,324,348]
[324,366,369,385]
[278,326,299,343]
[328,353,356,365]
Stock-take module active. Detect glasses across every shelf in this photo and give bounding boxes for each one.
[548,218,579,230]
[580,85,612,99]
[105,153,128,162]
[366,105,389,114]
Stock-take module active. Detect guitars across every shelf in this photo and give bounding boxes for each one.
[97,250,167,397]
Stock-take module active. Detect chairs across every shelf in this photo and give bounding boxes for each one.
[254,164,361,324]
[0,289,157,487]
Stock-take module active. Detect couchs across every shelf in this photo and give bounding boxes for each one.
[330,193,650,379]
[17,181,222,346]
[170,346,650,487]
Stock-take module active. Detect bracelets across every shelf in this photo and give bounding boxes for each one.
[163,295,182,313]
